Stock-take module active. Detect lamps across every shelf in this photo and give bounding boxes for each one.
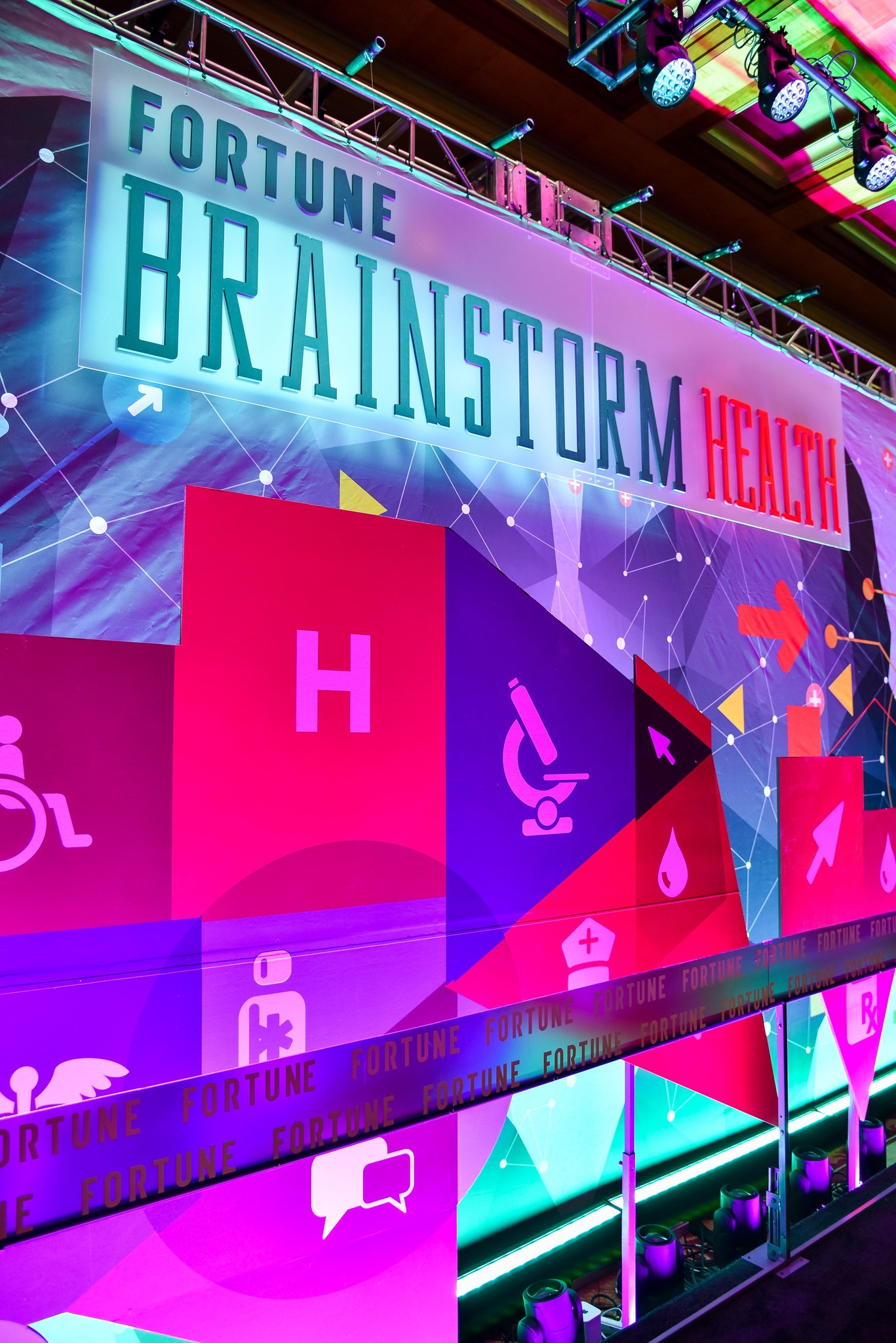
[636,0,696,111]
[517,1277,602,1343]
[712,1181,767,1269]
[845,1116,886,1182]
[852,114,896,192]
[757,34,809,123]
[788,1145,832,1224]
[635,1224,684,1296]
